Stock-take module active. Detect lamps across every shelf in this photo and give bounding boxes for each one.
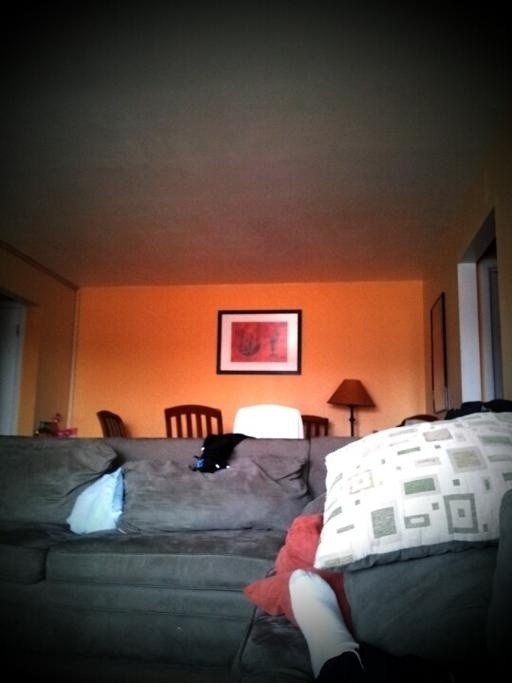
[327,379,373,437]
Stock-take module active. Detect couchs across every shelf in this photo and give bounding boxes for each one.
[1,433,512,683]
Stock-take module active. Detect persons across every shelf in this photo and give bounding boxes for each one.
[287,567,369,683]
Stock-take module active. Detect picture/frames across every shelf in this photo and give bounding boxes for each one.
[217,310,302,375]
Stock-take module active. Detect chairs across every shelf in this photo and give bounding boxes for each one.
[97,410,126,437]
[164,404,223,437]
[302,414,328,438]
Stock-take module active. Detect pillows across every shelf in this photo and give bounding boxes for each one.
[121,457,311,532]
[314,413,512,575]
